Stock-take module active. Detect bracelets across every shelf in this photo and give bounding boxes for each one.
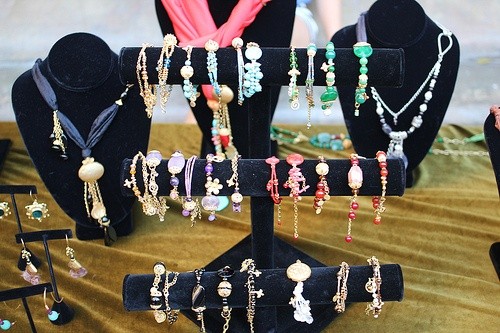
[124,33,388,333]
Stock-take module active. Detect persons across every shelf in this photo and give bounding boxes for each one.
[292,1,346,47]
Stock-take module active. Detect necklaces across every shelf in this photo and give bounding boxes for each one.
[163,0,268,162]
[356,11,453,169]
[32,58,134,246]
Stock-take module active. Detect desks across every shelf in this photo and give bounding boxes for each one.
[0,119,500,333]
